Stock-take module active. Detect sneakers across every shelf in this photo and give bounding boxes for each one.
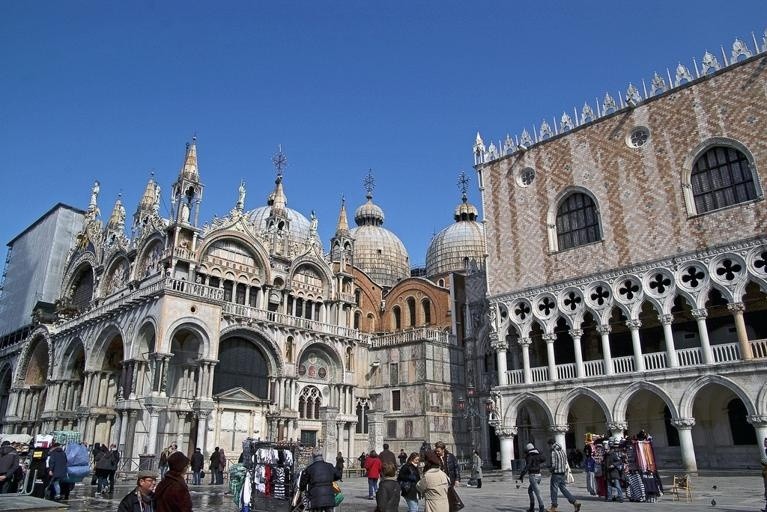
[574,501,581,512]
[547,507,558,512]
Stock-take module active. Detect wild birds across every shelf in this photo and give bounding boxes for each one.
[711,499,716,507]
[516,485,521,489]
[515,480,518,484]
[712,485,717,491]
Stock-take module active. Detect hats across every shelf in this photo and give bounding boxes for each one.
[138,470,158,479]
[424,451,441,465]
[169,452,190,472]
[526,443,535,449]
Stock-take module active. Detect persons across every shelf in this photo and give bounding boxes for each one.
[761,438,767,511]
[236,178,246,204]
[89,180,100,205]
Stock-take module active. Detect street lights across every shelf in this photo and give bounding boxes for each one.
[457,380,492,466]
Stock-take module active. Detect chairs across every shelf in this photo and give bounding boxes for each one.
[671,473,694,504]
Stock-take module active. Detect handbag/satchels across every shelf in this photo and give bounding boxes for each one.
[200,471,205,478]
[448,486,464,512]
[468,477,478,486]
[565,464,574,484]
[617,463,624,471]
[400,481,411,492]
